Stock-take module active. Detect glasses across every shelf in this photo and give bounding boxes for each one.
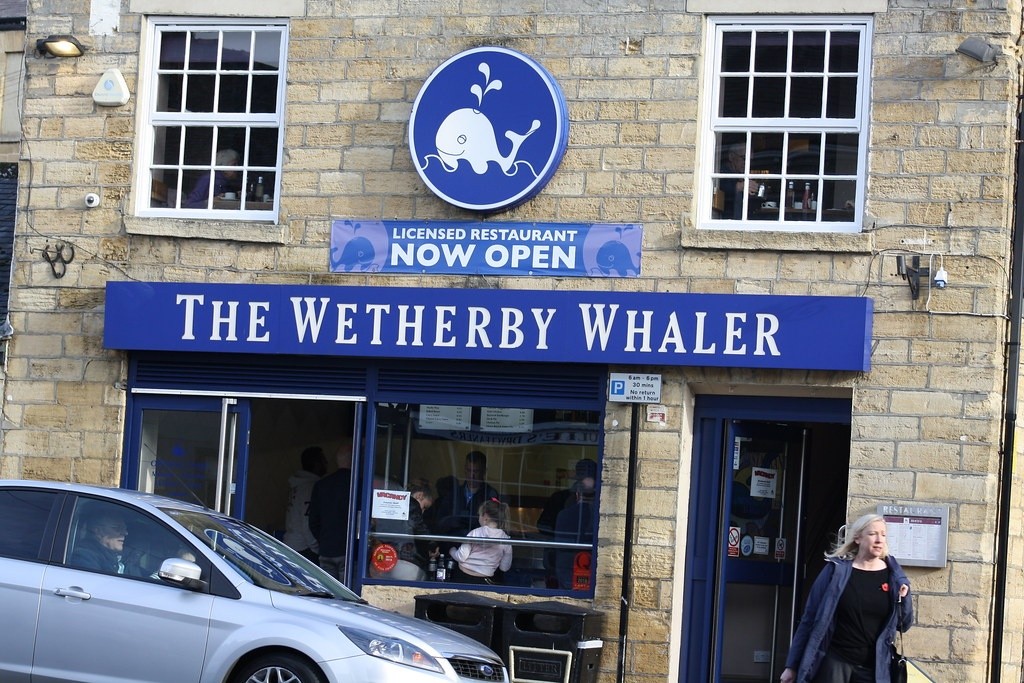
[466,468,481,473]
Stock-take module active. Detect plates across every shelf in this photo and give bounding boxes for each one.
[221,197,238,201]
[826,208,847,211]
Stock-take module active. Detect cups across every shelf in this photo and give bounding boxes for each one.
[811,201,816,210]
[760,201,777,207]
[755,184,768,201]
[223,192,236,199]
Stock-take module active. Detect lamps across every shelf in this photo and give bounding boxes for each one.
[36,35,85,58]
[955,35,998,61]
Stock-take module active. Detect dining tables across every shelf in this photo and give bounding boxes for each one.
[214,198,273,210]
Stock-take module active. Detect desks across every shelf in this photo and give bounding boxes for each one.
[759,207,853,223]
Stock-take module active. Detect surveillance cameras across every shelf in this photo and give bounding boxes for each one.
[85,193,99,207]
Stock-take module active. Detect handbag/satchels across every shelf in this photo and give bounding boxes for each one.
[890,645,907,683]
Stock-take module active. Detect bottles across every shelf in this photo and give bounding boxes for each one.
[786,181,795,208]
[254,177,264,202]
[802,182,811,210]
[428,554,454,582]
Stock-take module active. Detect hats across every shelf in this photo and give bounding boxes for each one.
[301,446,322,472]
[575,459,597,477]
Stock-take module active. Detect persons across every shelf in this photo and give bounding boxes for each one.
[368,475,441,562]
[71,508,196,595]
[537,459,597,590]
[721,143,765,218]
[427,452,499,550]
[309,441,364,585]
[282,446,328,567]
[449,499,513,584]
[184,149,240,209]
[780,515,914,683]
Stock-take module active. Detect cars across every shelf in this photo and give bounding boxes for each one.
[0,478,510,682]
